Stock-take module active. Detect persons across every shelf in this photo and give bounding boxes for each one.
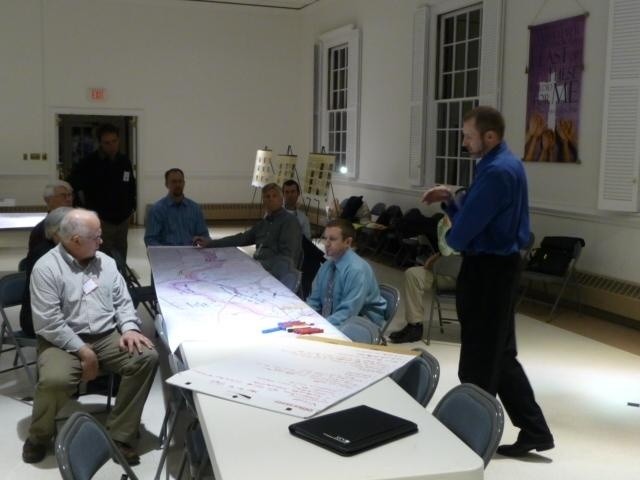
[538,129,554,162]
[144,169,211,247]
[390,189,469,342]
[525,114,545,160]
[555,117,578,162]
[20,206,75,338]
[437,106,555,455]
[193,183,303,269]
[67,122,137,278]
[22,208,160,463]
[306,220,387,345]
[20,180,74,248]
[282,180,311,239]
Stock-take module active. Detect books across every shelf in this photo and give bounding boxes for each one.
[288,405,419,456]
[420,185,465,215]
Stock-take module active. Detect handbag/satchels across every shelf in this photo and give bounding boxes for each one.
[527,244,571,275]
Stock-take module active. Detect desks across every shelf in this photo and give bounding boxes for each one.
[143,244,485,480]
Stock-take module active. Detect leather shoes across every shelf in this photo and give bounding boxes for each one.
[495,428,555,458]
[22,438,48,465]
[111,440,141,466]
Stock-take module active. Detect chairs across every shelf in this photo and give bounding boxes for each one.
[108,245,161,338]
[339,196,445,272]
[53,409,141,479]
[153,349,210,480]
[387,348,440,408]
[373,282,401,346]
[175,418,210,480]
[31,336,116,416]
[514,233,587,324]
[294,249,305,300]
[518,230,534,260]
[430,381,505,469]
[423,254,463,347]
[0,256,42,389]
[150,311,172,354]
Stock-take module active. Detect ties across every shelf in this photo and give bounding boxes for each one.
[321,265,336,318]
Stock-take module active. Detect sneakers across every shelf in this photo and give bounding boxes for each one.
[388,321,424,344]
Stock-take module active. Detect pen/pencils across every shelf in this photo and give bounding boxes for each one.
[262,320,323,335]
